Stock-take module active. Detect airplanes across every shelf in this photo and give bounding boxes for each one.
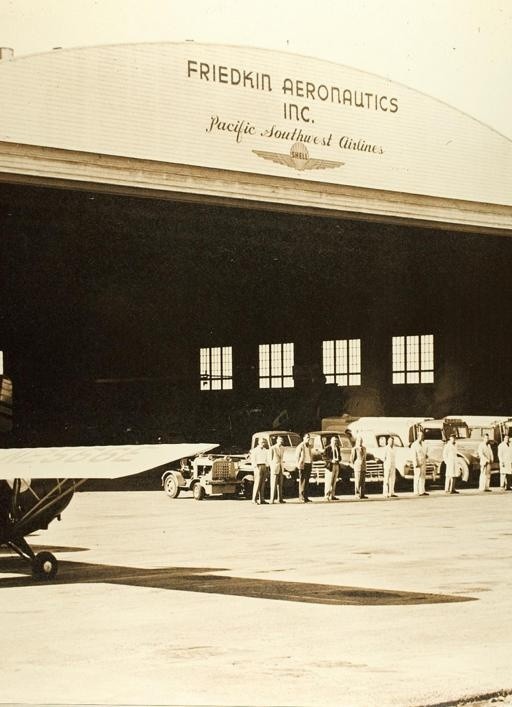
[0,442,222,581]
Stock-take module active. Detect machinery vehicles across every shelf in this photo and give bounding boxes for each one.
[160,453,242,500]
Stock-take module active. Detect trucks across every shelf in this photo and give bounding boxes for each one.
[322,415,512,489]
[195,431,303,499]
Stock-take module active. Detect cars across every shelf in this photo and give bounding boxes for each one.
[303,431,438,491]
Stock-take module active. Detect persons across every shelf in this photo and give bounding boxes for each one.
[497,435,512,491]
[269,437,286,504]
[295,433,312,504]
[443,433,459,495]
[410,432,430,496]
[350,436,368,500]
[382,437,398,498]
[478,433,494,493]
[250,437,268,505]
[322,437,342,503]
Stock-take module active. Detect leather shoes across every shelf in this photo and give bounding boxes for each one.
[252,487,512,505]
